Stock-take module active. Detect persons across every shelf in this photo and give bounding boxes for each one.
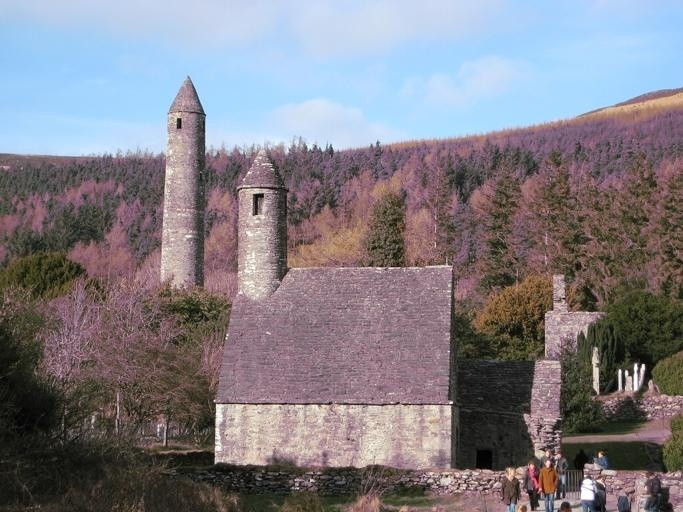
[594,450,609,470]
[579,471,674,512]
[499,448,572,512]
